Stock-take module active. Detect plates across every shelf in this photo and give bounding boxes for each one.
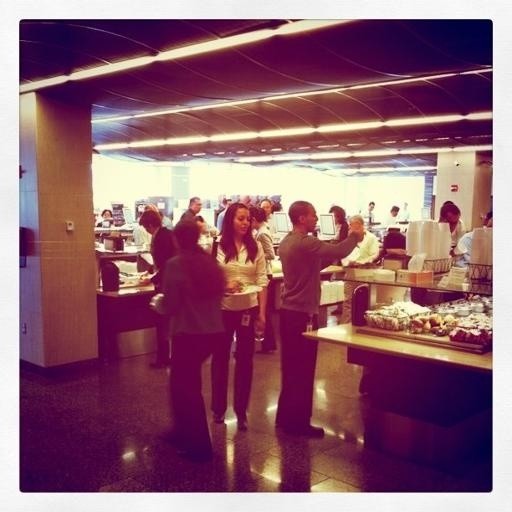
[376,270,396,282]
[228,285,264,294]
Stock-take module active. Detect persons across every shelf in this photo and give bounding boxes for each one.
[275,199,363,437]
[138,210,177,370]
[249,205,278,355]
[205,201,270,431]
[160,220,225,464]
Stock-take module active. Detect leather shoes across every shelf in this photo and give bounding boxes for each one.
[236,413,249,430]
[213,411,226,423]
[276,420,325,437]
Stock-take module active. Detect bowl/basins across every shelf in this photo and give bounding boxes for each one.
[404,219,451,260]
[436,298,493,314]
[470,228,493,264]
[119,272,150,282]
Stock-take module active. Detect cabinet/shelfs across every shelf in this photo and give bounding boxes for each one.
[303,266,492,473]
[96,245,170,359]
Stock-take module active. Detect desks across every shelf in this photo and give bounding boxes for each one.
[271,264,346,338]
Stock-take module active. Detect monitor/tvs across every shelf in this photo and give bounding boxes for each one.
[273,212,290,233]
[319,213,336,235]
